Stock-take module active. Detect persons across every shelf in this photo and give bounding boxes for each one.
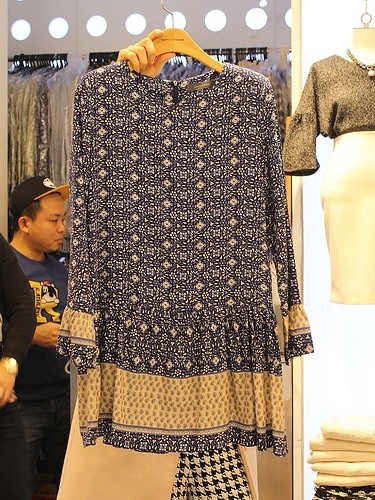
[54,30,255,500]
[5,175,71,491]
[1,232,39,500]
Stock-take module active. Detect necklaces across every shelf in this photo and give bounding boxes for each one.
[345,47,375,78]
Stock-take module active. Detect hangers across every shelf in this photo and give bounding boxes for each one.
[6,0,272,94]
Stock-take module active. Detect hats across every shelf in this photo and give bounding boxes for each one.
[10,176,69,218]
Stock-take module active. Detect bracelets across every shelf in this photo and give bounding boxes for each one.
[1,356,18,373]
